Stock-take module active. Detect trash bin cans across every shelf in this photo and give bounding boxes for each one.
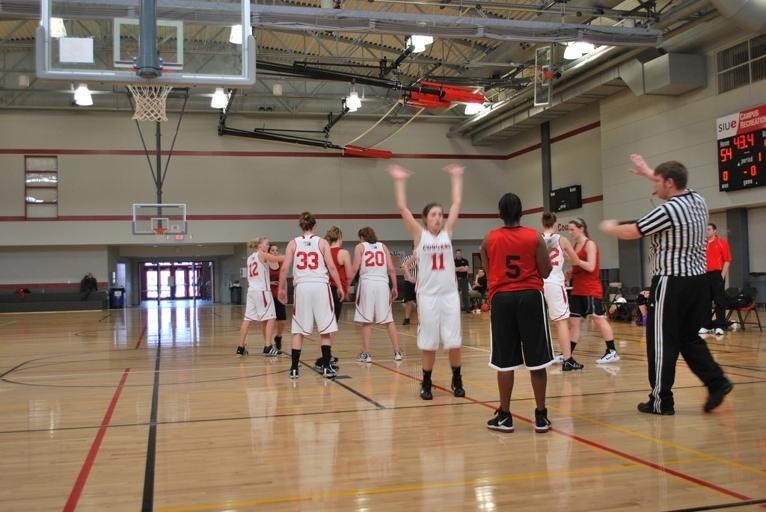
[109,288,124,309]
[230,287,241,305]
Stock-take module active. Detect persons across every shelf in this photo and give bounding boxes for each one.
[699,222,733,337]
[553,218,622,366]
[473,192,555,433]
[636,287,650,322]
[468,268,487,301]
[349,225,407,363]
[313,224,353,373]
[79,271,100,302]
[536,209,586,371]
[400,244,418,326]
[262,244,286,356]
[599,149,732,417]
[233,235,287,358]
[382,161,468,399]
[453,248,471,315]
[276,211,345,378]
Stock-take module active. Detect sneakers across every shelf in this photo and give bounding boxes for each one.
[355,351,372,363]
[393,351,403,361]
[563,358,584,370]
[552,353,565,364]
[450,374,465,397]
[595,349,621,364]
[702,378,733,414]
[534,407,551,432]
[263,336,283,355]
[236,346,249,357]
[639,403,675,415]
[403,317,411,325]
[487,410,514,432]
[420,380,433,400]
[698,327,725,335]
[289,357,339,378]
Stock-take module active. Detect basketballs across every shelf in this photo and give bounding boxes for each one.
[481,304,490,312]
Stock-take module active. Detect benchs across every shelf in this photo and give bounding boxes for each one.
[0,286,110,314]
